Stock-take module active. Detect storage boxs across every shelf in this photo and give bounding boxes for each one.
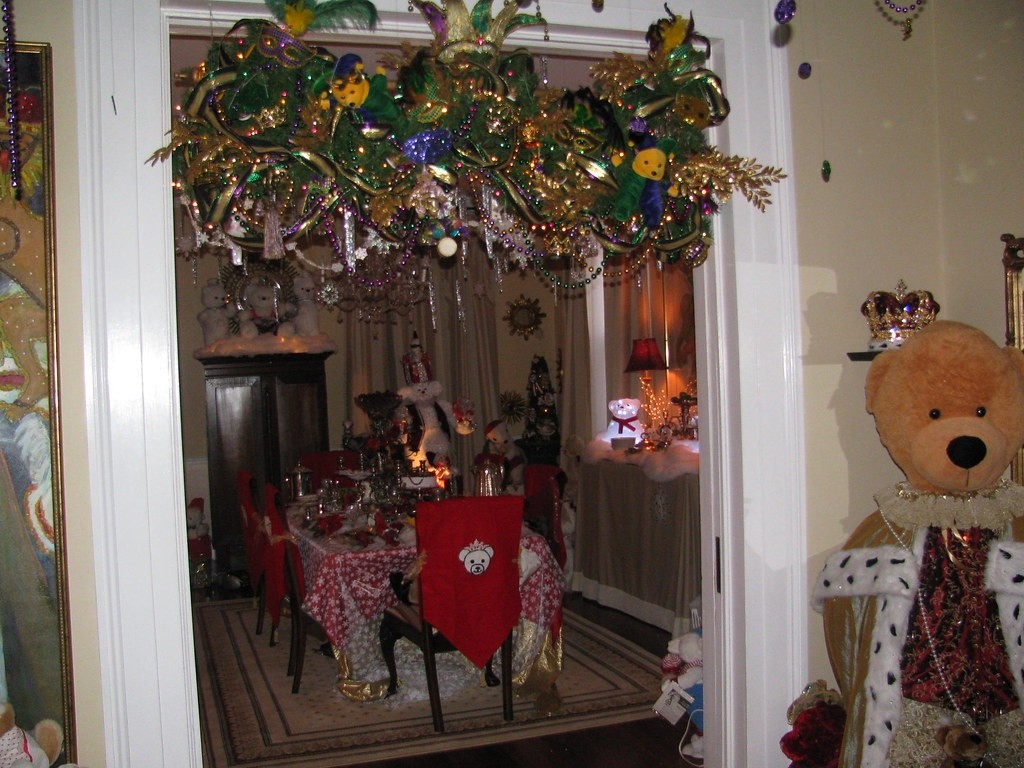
[401,476,436,488]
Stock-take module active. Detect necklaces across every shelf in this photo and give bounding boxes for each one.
[877,505,1011,768]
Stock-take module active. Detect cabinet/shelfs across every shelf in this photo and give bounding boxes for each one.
[196,351,334,592]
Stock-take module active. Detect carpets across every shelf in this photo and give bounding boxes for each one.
[194,595,665,767]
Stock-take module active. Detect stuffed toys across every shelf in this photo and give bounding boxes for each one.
[564,435,583,457]
[394,381,482,469]
[186,497,212,561]
[237,276,321,337]
[646,427,660,439]
[812,321,1024,768]
[0,704,64,768]
[579,397,654,468]
[662,625,705,759]
[483,420,526,483]
[197,279,237,345]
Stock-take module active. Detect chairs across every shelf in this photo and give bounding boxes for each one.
[236,450,564,732]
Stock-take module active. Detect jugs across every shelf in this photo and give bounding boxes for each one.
[285,461,314,502]
[471,459,504,496]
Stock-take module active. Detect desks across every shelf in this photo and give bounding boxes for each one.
[285,488,571,702]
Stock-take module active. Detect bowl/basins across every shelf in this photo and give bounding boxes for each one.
[612,438,635,450]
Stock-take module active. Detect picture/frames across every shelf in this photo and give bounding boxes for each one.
[0,42,81,768]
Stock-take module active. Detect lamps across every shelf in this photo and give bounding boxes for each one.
[621,334,669,451]
